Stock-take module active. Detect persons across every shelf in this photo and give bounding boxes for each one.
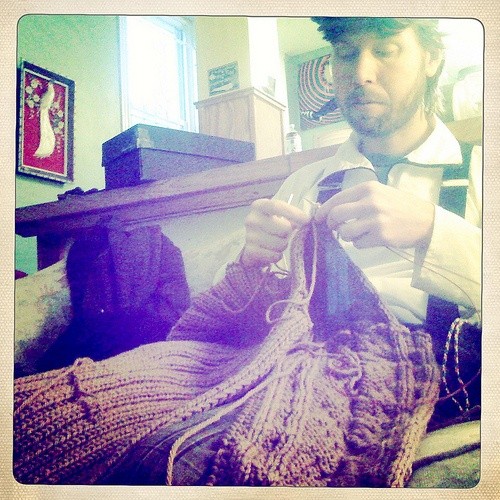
[214,15,482,434]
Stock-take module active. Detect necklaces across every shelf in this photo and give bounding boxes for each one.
[369,161,397,169]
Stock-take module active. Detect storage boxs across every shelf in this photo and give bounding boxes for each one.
[102,124,256,194]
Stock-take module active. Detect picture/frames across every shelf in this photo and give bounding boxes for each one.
[16,61,76,185]
